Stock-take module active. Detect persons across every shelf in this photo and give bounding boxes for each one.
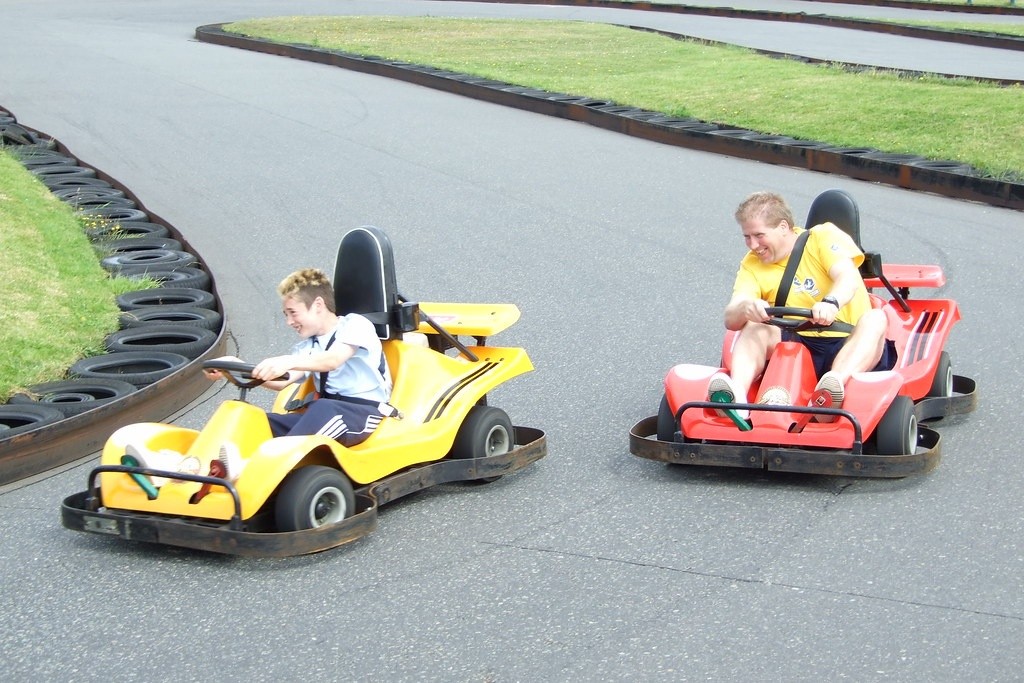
[200,268,392,448]
[708,191,887,424]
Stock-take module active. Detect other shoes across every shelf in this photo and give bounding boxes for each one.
[219,444,241,483]
[808,371,844,423]
[709,374,749,419]
[127,445,160,487]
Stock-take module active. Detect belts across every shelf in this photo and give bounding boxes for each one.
[325,395,380,407]
